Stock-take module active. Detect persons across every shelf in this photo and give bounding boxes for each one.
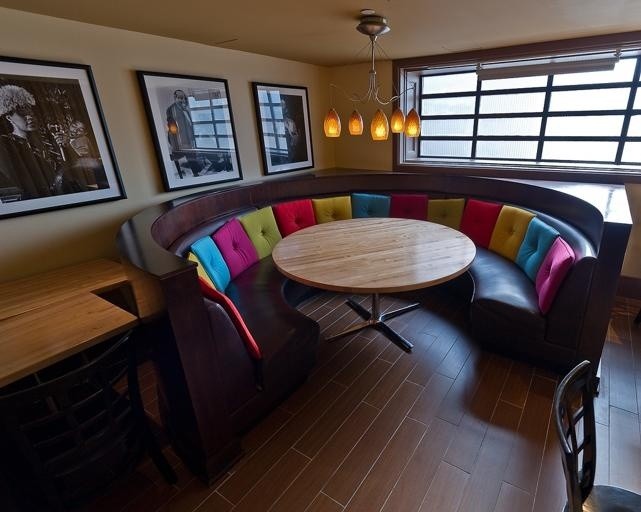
[275,97,299,162]
[0,84,57,200]
[166,90,199,166]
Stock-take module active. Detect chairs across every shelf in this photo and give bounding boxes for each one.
[7,329,188,512]
[553,358,640,511]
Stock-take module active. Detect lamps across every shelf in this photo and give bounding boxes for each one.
[323,14,427,144]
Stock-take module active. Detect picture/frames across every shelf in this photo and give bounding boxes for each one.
[132,65,245,191]
[253,76,316,174]
[1,52,129,212]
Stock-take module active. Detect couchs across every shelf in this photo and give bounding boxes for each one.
[115,167,633,487]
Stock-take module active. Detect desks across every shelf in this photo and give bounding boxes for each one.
[272,212,477,355]
[1,293,138,386]
[0,255,137,318]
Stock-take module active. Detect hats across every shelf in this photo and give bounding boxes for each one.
[0,85,35,116]
